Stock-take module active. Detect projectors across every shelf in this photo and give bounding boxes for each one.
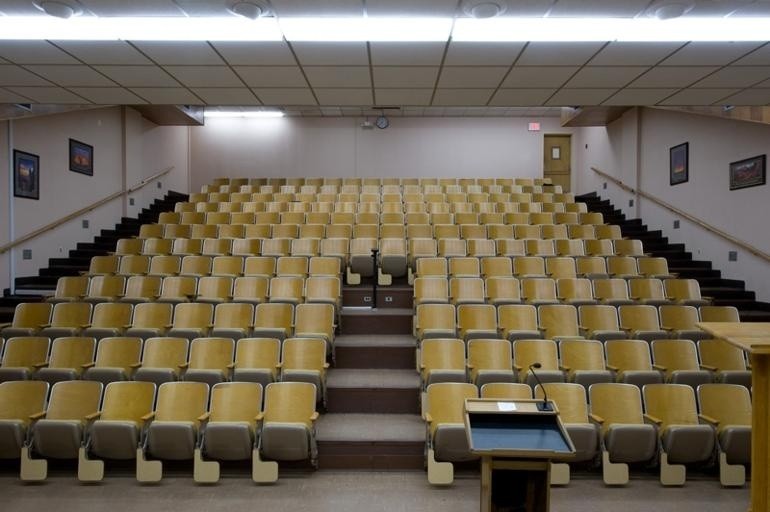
[359,121,374,129]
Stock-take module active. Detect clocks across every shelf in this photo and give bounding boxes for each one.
[374,115,390,129]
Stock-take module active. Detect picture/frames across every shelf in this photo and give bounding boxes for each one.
[669,142,689,187]
[69,138,93,177]
[730,155,767,193]
[12,148,40,201]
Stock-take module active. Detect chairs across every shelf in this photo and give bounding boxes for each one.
[87,175,671,276]
[0,274,340,484]
[414,277,751,488]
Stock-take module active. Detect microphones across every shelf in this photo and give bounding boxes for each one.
[528,363,554,411]
